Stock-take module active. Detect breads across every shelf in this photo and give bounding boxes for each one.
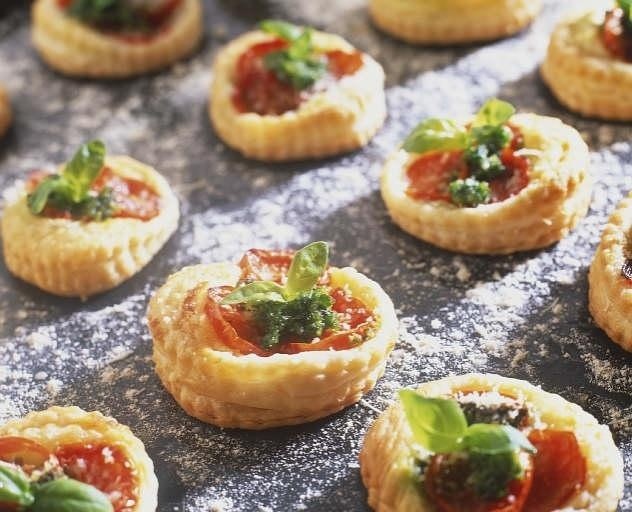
[29,0,203,77]
[540,0,632,122]
[360,373,624,512]
[0,140,181,297]
[209,19,387,165]
[0,85,12,139]
[369,0,542,47]
[588,189,632,354]
[379,97,591,256]
[145,240,399,433]
[0,406,159,512]
